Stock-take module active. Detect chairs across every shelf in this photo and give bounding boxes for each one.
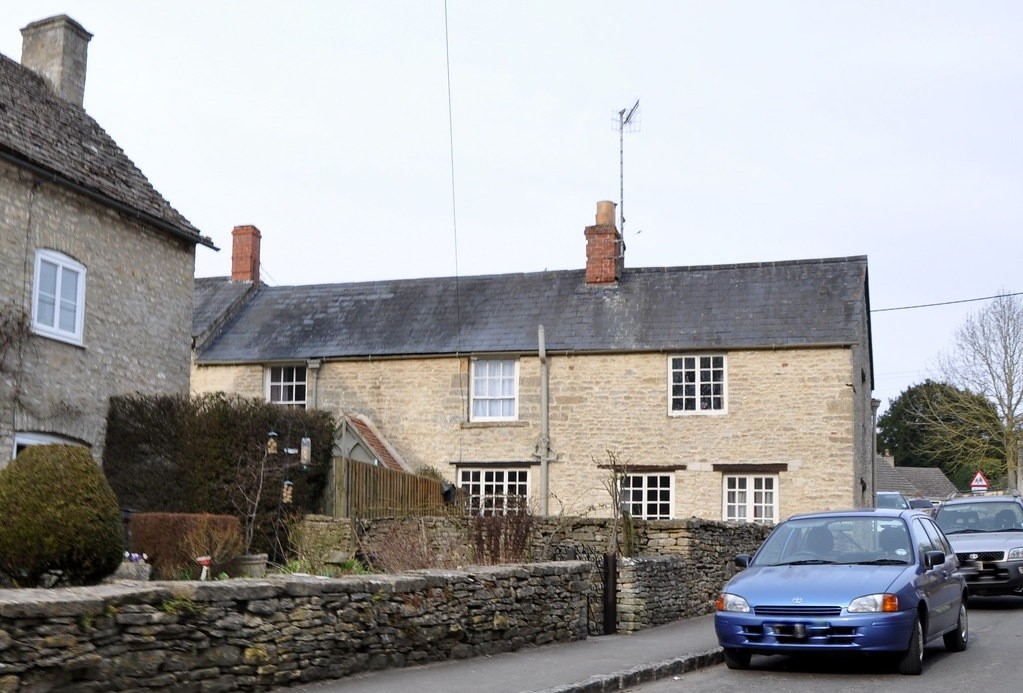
[995,509,1017,529]
[805,526,835,561]
[877,528,911,561]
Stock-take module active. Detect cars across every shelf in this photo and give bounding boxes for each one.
[875,492,911,510]
[909,498,937,516]
[713,509,970,675]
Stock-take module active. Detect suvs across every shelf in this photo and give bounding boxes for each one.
[925,490,1023,598]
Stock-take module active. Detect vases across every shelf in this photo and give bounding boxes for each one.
[234,554,268,578]
